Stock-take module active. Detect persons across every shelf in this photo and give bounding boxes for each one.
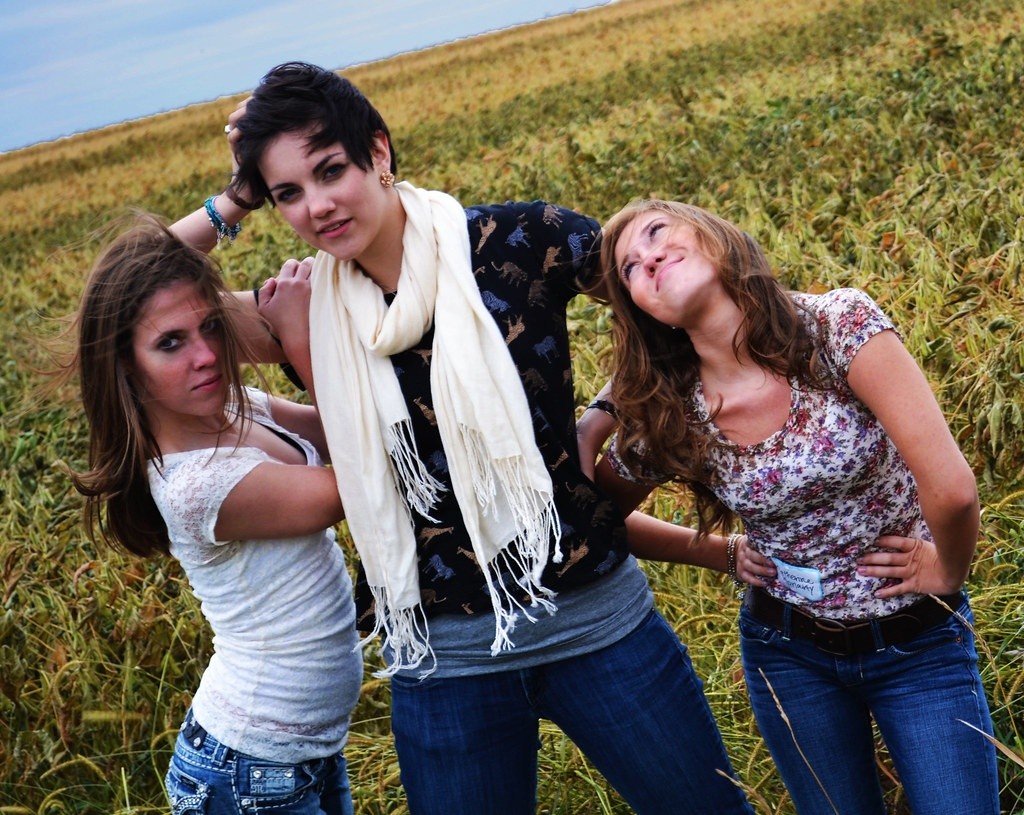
[593,200,1000,815]
[159,62,751,815]
[62,215,363,815]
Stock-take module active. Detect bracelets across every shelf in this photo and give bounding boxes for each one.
[225,185,266,210]
[585,400,621,419]
[728,533,744,600]
[204,194,240,246]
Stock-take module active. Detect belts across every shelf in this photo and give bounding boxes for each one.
[744,585,966,655]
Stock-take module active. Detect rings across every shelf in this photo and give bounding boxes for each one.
[224,123,238,133]
